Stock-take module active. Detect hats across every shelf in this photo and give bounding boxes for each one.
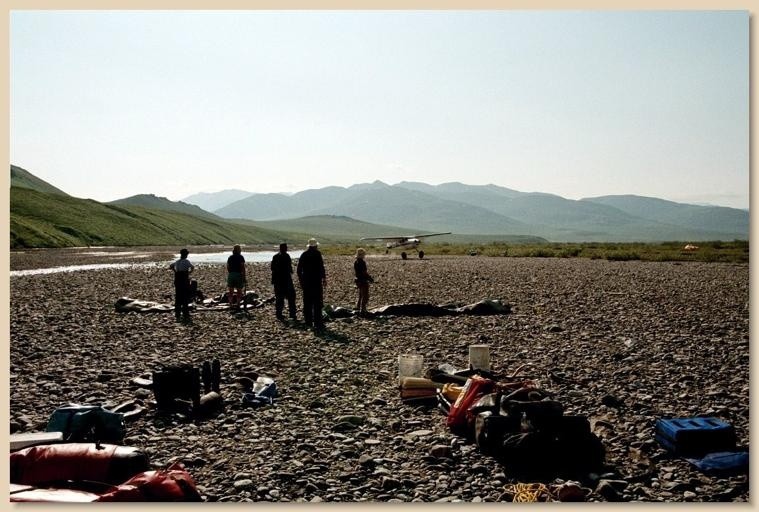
[306,238,320,247]
[180,248,189,255]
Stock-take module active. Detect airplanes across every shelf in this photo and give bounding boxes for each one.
[360,232,452,260]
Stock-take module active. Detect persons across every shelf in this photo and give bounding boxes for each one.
[227,244,246,310]
[354,248,375,313]
[296,238,330,326]
[169,248,195,320]
[271,243,297,320]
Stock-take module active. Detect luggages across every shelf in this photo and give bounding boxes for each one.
[655,417,738,453]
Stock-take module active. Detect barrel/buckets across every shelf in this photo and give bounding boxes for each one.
[398,355,423,382]
[469,345,489,371]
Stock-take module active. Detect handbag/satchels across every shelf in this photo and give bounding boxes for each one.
[437,371,590,465]
